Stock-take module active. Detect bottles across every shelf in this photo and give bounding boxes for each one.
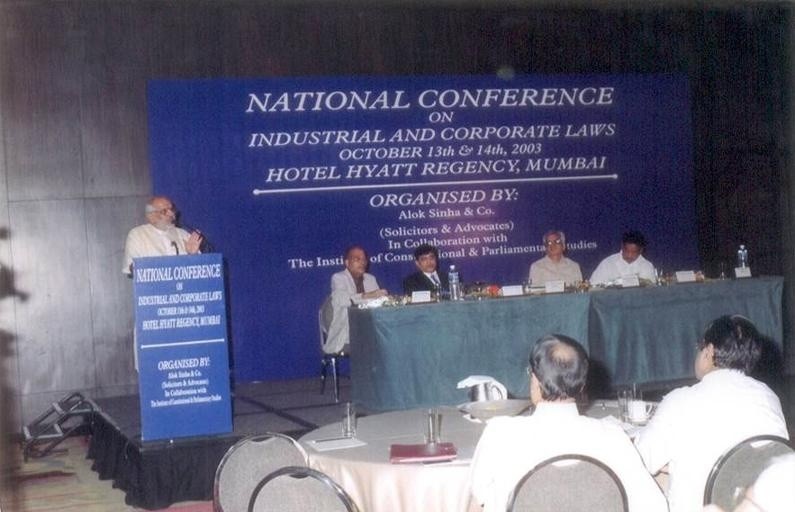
[737,244,748,268]
[448,264,461,301]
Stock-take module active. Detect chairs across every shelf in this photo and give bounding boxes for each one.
[213,431,358,512]
[507,454,629,511]
[702,435,795,512]
[318,295,348,403]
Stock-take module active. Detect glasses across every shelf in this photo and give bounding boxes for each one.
[546,238,562,246]
[148,207,176,215]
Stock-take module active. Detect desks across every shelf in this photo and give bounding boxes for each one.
[295,400,670,512]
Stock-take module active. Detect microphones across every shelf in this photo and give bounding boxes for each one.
[175,220,216,252]
[171,241,179,255]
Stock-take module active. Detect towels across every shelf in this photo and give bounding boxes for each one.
[456,370,508,401]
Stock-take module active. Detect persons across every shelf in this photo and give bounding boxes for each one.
[324,246,390,359]
[525,231,583,287]
[468,333,667,511]
[120,198,203,374]
[591,230,660,285]
[632,316,790,510]
[404,244,460,293]
[699,454,795,511]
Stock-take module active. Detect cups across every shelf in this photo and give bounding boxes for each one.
[618,390,633,420]
[422,409,441,446]
[655,266,664,285]
[629,401,653,426]
[522,277,532,294]
[341,401,357,439]
[434,284,444,301]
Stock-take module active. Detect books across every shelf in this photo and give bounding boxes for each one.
[388,441,460,464]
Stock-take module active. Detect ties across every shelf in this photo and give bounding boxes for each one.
[431,274,437,282]
